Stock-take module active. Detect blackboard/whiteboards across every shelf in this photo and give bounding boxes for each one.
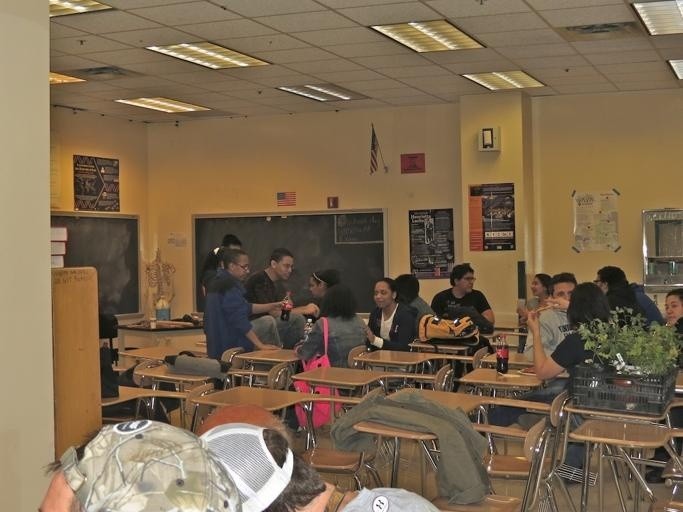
[72,154,120,212]
[409,208,455,280]
[50,210,145,319]
[191,208,388,318]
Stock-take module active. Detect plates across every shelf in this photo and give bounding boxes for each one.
[517,368,538,376]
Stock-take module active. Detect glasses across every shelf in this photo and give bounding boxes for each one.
[231,261,248,270]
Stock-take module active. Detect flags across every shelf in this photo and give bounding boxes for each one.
[276,192,297,208]
[368,127,379,177]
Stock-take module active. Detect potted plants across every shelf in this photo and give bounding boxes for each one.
[581,308,680,404]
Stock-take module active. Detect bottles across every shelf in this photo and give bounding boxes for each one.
[303,319,314,345]
[649,260,655,274]
[280,291,291,321]
[495,333,509,375]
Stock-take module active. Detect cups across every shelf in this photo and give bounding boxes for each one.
[668,261,676,274]
[150,318,156,329]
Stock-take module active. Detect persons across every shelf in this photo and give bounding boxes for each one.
[645,288,683,483]
[198,423,440,511]
[199,233,340,391]
[274,283,372,437]
[395,273,436,337]
[430,263,496,393]
[469,264,666,487]
[362,278,418,385]
[425,219,433,243]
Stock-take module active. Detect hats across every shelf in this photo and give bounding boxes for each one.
[198,422,294,512]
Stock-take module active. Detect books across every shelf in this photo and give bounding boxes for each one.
[51,224,68,269]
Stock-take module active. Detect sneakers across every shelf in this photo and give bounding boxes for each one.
[645,470,665,483]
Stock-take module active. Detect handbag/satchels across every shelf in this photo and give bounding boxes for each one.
[294,354,344,428]
[163,351,232,377]
[418,312,480,347]
